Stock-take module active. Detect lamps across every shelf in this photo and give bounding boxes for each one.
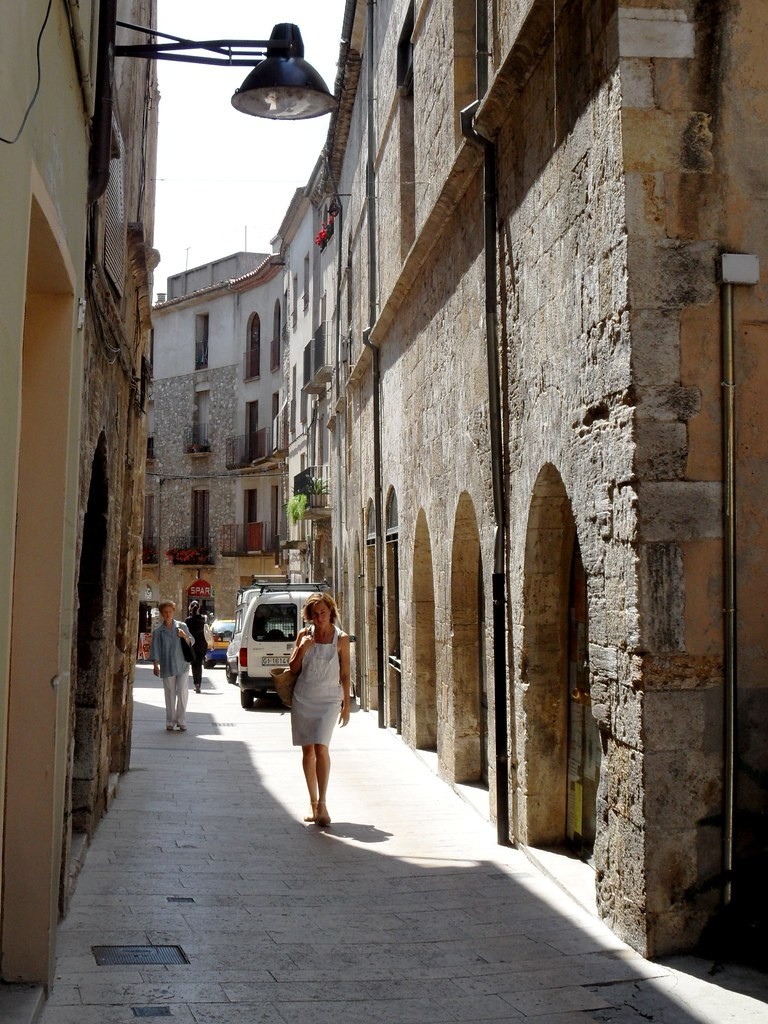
[89,0,340,204]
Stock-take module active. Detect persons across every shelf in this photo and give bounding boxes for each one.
[182,600,215,694]
[270,592,350,826]
[149,600,195,732]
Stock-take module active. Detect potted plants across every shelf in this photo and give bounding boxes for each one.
[306,473,330,507]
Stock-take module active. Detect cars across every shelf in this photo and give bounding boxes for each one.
[204,619,239,668]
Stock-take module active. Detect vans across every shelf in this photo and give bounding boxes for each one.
[225,582,346,708]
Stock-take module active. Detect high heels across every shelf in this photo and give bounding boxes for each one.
[303,801,331,827]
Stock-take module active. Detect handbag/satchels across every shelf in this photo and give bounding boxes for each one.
[179,621,194,662]
[269,624,312,707]
[203,614,215,651]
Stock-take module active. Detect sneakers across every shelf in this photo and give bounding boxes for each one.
[166,726,173,731]
[176,723,187,731]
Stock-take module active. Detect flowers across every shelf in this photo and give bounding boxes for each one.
[315,214,334,249]
[166,546,211,565]
[143,545,160,563]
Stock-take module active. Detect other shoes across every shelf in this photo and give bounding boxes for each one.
[193,684,200,693]
